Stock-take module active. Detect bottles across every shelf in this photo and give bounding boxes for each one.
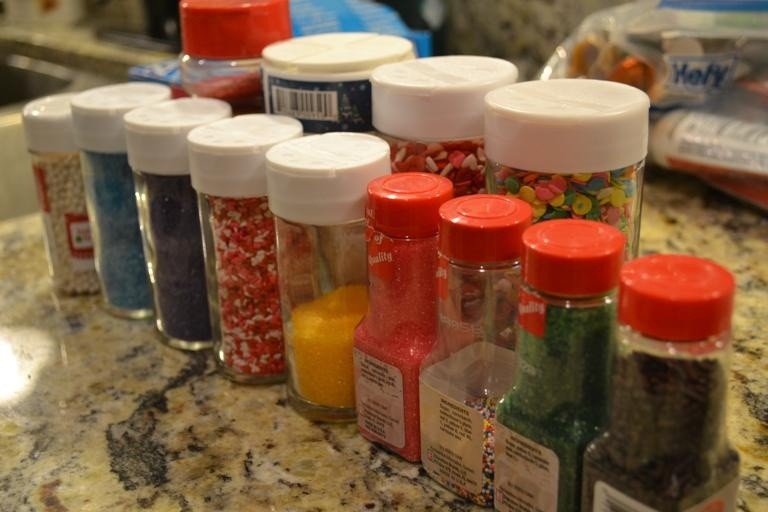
[264,130,392,423]
[185,114,303,387]
[348,171,453,463]
[160,0,652,253]
[579,254,740,511]
[18,89,98,296]
[121,94,234,353]
[71,80,177,321]
[419,189,536,504]
[491,219,625,510]
